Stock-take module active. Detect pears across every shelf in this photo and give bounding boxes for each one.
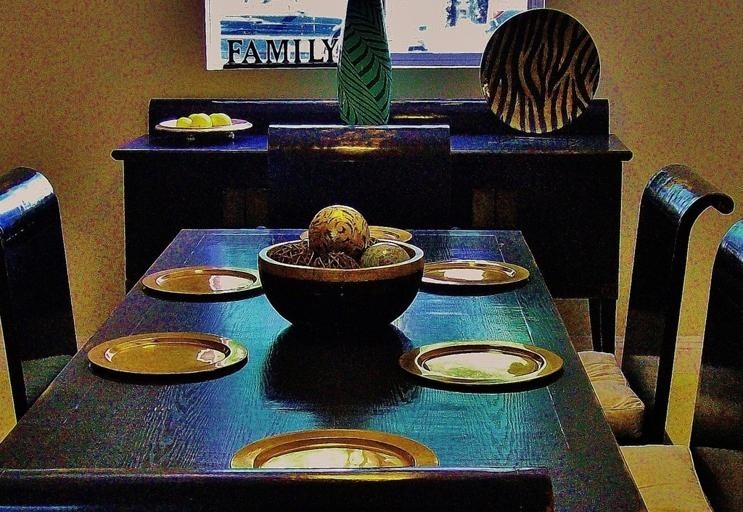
[176,112,232,128]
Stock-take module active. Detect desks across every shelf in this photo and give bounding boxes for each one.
[106,96,632,355]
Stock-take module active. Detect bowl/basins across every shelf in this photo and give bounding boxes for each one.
[257,239,423,331]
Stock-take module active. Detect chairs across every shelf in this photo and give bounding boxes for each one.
[570,163,732,443]
[1,166,79,424]
[614,213,742,511]
[0,468,553,511]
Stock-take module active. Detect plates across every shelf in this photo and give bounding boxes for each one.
[141,265,263,297]
[230,428,439,471]
[298,225,413,241]
[154,114,251,135]
[396,337,564,387]
[479,7,601,136]
[86,329,247,376]
[422,260,531,289]
